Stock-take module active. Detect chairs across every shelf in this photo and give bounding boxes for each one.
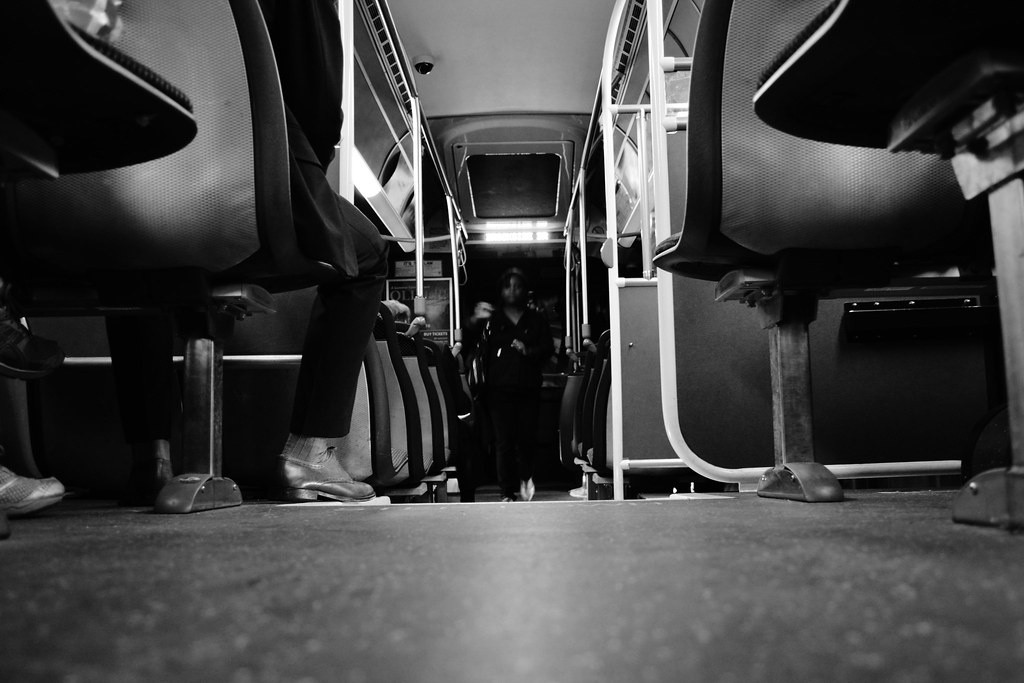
[0,1,1024,528]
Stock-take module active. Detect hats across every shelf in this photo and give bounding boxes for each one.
[495,267,527,289]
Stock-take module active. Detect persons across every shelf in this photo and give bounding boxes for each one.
[0,286,67,517]
[105,0,474,501]
[569,347,596,499]
[481,266,553,501]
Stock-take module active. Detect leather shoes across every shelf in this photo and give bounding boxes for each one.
[140,461,174,507]
[278,446,377,503]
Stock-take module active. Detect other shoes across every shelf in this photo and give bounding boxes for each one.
[0,466,65,515]
[569,473,630,499]
[698,473,726,493]
[520,477,535,501]
[0,280,66,379]
[644,468,684,495]
[502,496,519,503]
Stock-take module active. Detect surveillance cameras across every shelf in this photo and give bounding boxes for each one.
[412,54,434,75]
